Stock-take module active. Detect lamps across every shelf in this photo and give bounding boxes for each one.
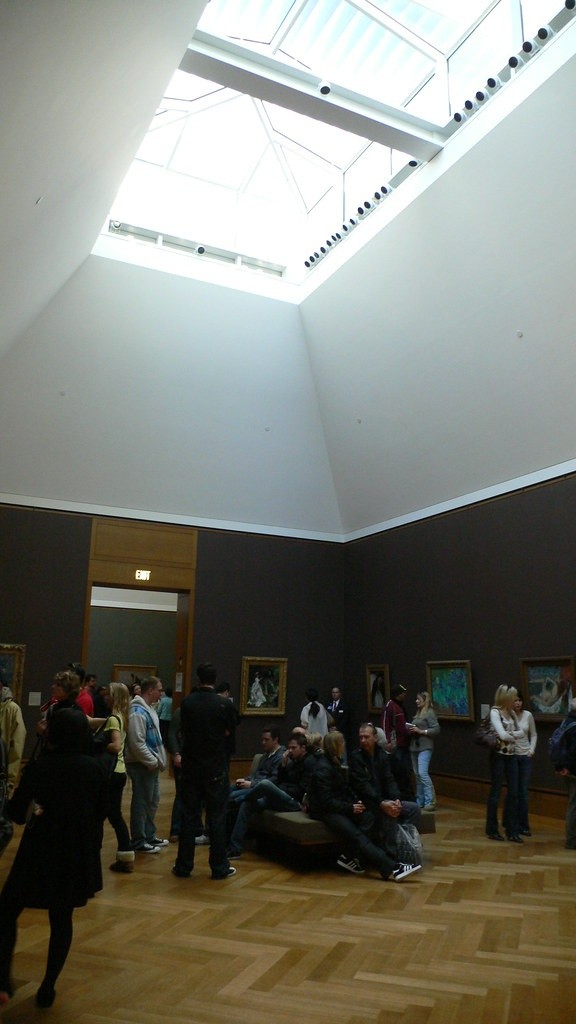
[195,243,207,257]
[407,159,423,169]
[563,1,576,14]
[453,26,556,126]
[304,185,396,272]
[317,80,331,97]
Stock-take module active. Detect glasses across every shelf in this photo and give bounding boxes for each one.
[505,682,514,695]
[68,662,77,676]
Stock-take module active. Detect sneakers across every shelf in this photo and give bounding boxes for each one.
[169,833,180,843]
[134,841,162,853]
[170,862,191,877]
[149,835,170,847]
[337,852,366,873]
[224,845,243,860]
[193,835,214,845]
[391,861,422,882]
[214,866,236,877]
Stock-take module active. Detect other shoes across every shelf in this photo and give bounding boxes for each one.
[36,988,57,1008]
[509,834,525,845]
[566,842,576,849]
[1,982,16,1006]
[423,803,438,811]
[520,827,533,837]
[489,832,505,841]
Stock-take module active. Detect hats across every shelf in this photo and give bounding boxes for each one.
[391,682,406,695]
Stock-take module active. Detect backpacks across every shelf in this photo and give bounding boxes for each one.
[547,717,575,770]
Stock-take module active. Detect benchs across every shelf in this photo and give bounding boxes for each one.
[246,753,436,875]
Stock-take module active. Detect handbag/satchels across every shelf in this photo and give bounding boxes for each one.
[390,822,424,868]
[0,778,14,860]
[93,713,122,776]
[473,706,508,752]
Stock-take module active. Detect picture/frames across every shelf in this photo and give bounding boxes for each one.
[518,656,576,724]
[424,659,474,723]
[111,663,163,688]
[0,642,28,707]
[238,656,288,716]
[365,662,392,716]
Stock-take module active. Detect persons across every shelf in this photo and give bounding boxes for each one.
[308,732,325,754]
[157,689,173,748]
[324,687,350,770]
[90,682,135,873]
[300,687,328,738]
[36,673,84,739]
[123,676,169,853]
[169,684,203,842]
[172,662,236,880]
[129,683,141,702]
[215,682,234,773]
[84,674,96,691]
[0,698,110,1008]
[52,663,93,718]
[307,732,422,882]
[486,683,525,843]
[380,683,412,775]
[291,727,310,744]
[409,690,440,811]
[347,721,420,858]
[502,688,537,836]
[0,667,26,800]
[196,725,286,845]
[555,697,576,849]
[226,732,314,860]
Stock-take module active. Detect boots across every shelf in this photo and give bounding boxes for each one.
[110,850,137,872]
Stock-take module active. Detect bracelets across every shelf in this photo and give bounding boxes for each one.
[424,729,427,735]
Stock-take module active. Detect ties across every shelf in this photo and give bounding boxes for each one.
[332,701,338,716]
